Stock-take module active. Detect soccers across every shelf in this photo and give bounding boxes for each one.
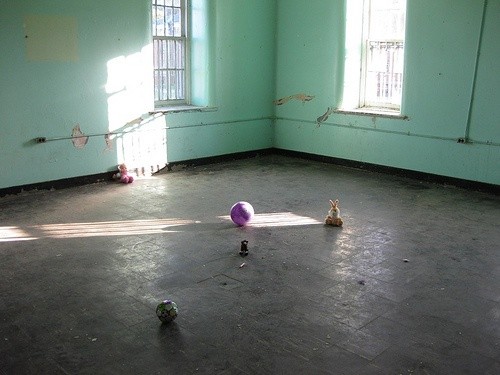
[156,300,178,323]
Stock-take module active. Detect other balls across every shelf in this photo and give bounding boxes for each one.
[230,201,254,226]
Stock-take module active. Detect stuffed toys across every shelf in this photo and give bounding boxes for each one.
[324,199,343,227]
